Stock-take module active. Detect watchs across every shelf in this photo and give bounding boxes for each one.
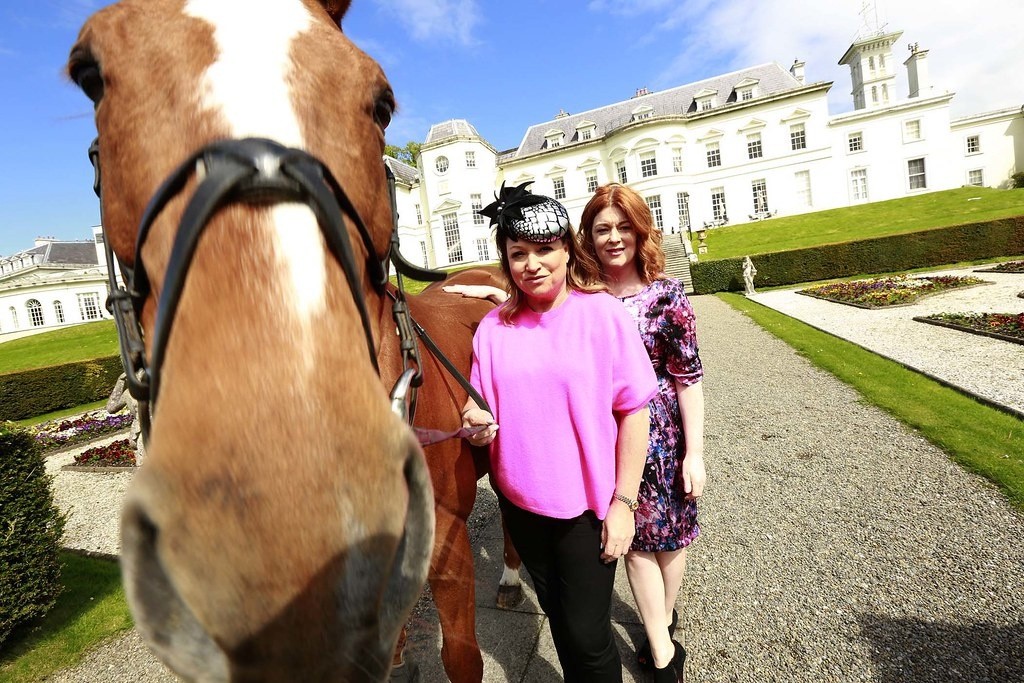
[613,491,639,511]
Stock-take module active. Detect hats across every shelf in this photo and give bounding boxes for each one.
[480,178,569,242]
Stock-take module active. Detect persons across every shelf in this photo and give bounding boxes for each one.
[106,348,152,469]
[742,256,757,295]
[575,182,705,683]
[462,180,658,683]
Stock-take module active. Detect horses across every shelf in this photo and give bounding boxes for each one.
[60,0,521,683]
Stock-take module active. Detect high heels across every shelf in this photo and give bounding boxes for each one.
[655,639,685,683]
[637,605,679,666]
